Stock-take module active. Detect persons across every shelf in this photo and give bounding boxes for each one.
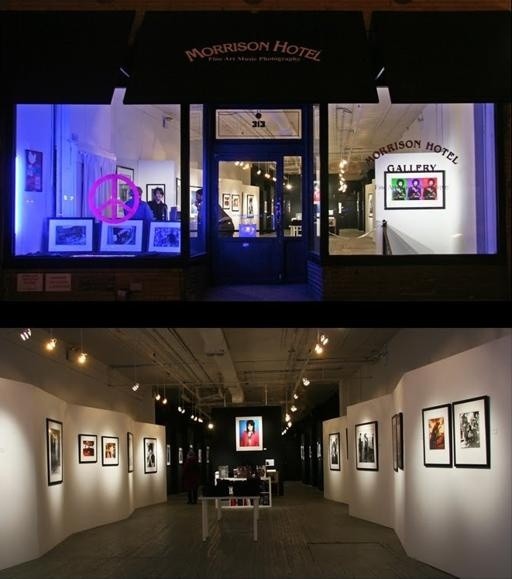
[393,180,405,201]
[331,442,339,465]
[193,189,233,236]
[358,433,374,462]
[423,179,436,199]
[240,420,259,447]
[123,187,155,221]
[147,443,156,468]
[147,188,168,220]
[408,179,421,200]
[183,452,202,505]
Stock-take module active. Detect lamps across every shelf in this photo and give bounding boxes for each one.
[230,154,294,195]
[18,328,88,366]
[129,358,215,431]
[279,329,329,429]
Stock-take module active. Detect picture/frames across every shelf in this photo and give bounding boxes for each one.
[45,217,181,255]
[236,416,264,452]
[452,395,490,469]
[421,402,454,468]
[383,170,446,209]
[24,148,44,192]
[328,412,405,474]
[367,193,375,219]
[45,419,156,486]
[107,164,255,220]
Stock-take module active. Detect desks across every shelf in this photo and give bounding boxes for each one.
[287,211,338,235]
[194,463,288,544]
